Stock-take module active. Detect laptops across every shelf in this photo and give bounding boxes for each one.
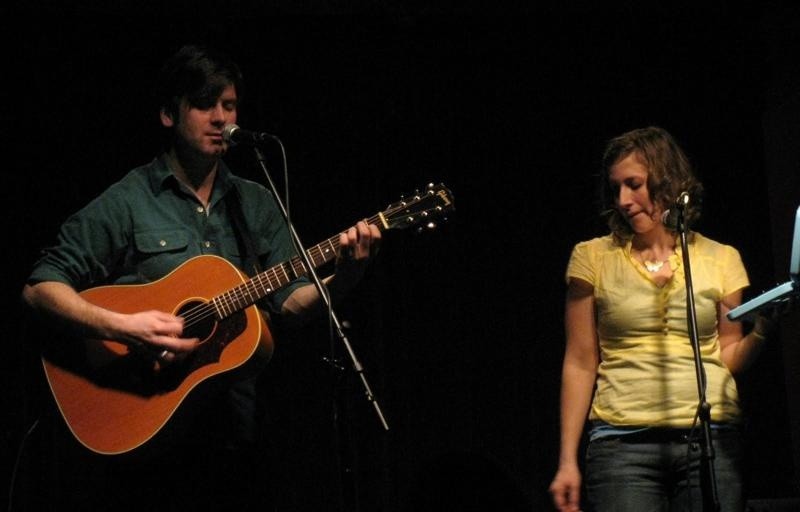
[727,203,800,322]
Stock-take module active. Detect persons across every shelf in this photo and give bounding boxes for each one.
[547,127,793,512]
[20,47,385,512]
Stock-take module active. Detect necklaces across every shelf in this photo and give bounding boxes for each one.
[635,240,675,272]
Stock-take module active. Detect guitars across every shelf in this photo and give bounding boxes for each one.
[42,182,457,456]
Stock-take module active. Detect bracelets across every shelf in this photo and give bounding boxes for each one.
[753,331,764,340]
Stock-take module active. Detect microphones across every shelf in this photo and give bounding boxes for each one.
[660,190,690,234]
[222,123,271,146]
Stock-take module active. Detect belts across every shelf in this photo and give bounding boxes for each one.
[620,428,739,443]
